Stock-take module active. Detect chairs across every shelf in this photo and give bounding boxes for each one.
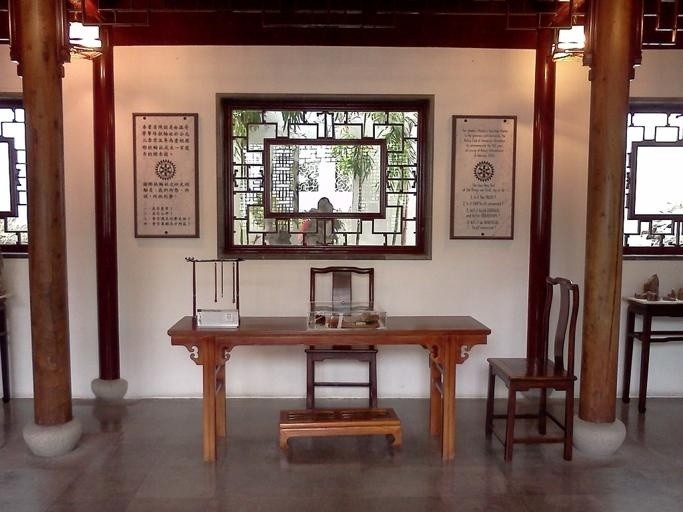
[303,265,380,407]
[485,275,582,462]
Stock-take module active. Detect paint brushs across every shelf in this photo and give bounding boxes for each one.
[215,261,235,304]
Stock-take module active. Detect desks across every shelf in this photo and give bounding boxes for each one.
[167,315,490,462]
[621,294,682,413]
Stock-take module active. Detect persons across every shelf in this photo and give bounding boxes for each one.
[296,197,339,246]
